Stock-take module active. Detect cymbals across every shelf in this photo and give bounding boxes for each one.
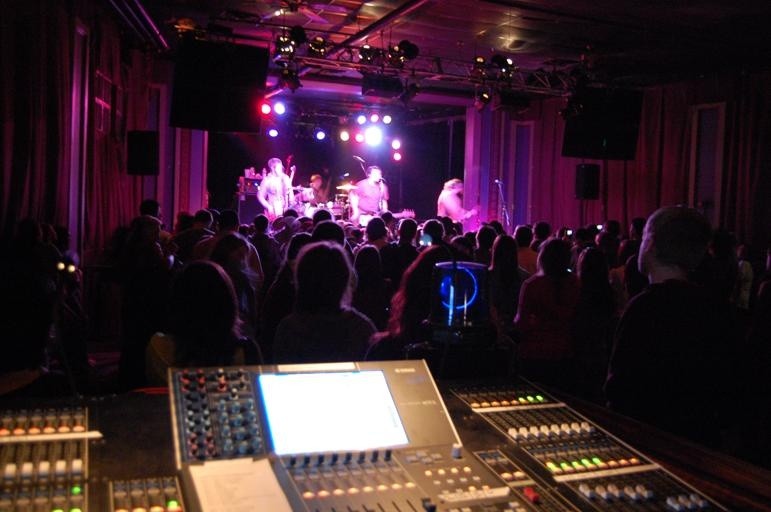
[336,186,358,190]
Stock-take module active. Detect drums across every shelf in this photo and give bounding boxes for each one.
[335,194,348,202]
[323,204,342,216]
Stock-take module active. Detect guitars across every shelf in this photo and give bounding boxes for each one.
[359,209,416,227]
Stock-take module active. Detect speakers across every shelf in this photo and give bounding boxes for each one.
[168,39,269,135]
[575,164,600,200]
[561,87,645,160]
[126,129,159,176]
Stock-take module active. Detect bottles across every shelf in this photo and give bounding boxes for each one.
[262,168,267,180]
[249,167,255,179]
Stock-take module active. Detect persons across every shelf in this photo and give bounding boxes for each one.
[349,165,393,224]
[300,174,329,207]
[256,158,296,217]
[437,178,481,225]
[2,198,771,467]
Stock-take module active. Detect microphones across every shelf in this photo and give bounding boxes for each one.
[495,178,502,184]
[352,155,365,163]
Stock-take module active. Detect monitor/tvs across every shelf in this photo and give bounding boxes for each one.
[256,372,408,455]
